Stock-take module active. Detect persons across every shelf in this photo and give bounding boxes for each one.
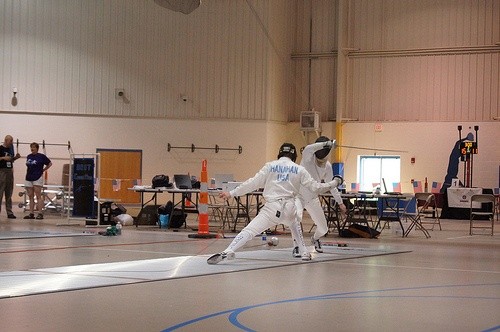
[24,142,52,219]
[207,143,344,264]
[292,136,347,257]
[0,134,21,219]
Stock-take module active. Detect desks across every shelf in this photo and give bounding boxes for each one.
[128,187,203,228]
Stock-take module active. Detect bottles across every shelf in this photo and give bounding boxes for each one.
[211,176,215,188]
[261,232,267,246]
[116,222,122,235]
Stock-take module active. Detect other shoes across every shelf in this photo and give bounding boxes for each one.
[292,246,301,257]
[36,213,43,219]
[302,251,312,261]
[206,249,236,265]
[24,213,35,219]
[310,236,324,253]
[8,214,16,218]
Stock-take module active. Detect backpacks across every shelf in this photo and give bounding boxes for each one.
[152,174,173,189]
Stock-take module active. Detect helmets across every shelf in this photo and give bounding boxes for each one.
[314,136,332,160]
[277,143,297,163]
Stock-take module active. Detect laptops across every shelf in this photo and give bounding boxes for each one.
[174,175,193,189]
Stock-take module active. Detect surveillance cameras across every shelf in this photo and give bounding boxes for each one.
[181,95,188,101]
[116,88,124,98]
[13,88,17,95]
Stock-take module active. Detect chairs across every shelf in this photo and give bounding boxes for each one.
[469,195,495,236]
[210,190,441,239]
